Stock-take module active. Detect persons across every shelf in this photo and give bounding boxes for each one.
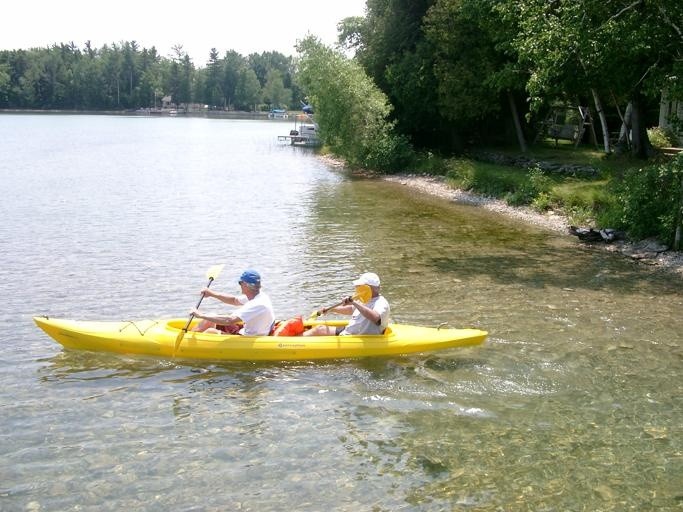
[298,272,389,337]
[190,270,276,337]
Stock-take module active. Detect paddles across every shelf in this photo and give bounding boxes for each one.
[172,264,223,358]
[308,285,372,320]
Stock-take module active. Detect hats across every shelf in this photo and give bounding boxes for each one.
[241,269,261,284]
[352,273,379,286]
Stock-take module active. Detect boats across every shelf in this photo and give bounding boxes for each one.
[32,311,489,363]
